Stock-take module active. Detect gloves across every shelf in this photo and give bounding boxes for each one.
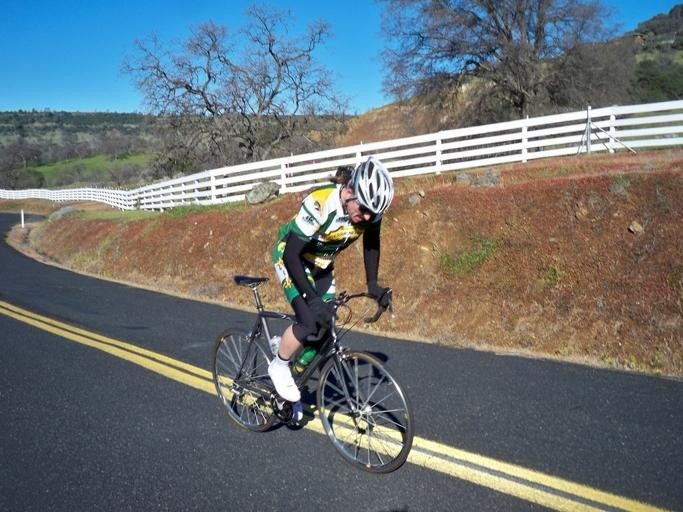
[375,286,393,309]
[305,295,339,329]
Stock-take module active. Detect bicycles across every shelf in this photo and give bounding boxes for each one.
[210,274,414,472]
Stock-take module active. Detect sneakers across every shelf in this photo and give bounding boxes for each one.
[290,400,304,422]
[267,357,302,403]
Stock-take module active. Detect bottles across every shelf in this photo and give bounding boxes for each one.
[291,345,315,377]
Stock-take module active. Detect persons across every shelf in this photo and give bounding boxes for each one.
[268,157,394,422]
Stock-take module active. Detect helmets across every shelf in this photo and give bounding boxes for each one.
[351,156,394,214]
